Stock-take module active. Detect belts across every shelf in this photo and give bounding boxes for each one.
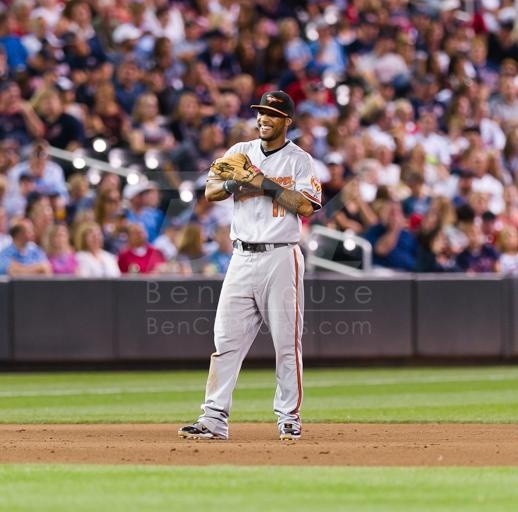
[233,242,297,253]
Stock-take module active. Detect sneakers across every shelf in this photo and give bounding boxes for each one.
[279,421,301,440]
[178,422,228,440]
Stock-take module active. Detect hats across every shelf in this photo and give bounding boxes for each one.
[250,90,296,118]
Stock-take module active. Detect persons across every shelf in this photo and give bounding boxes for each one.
[1,2,518,279]
[177,89,323,440]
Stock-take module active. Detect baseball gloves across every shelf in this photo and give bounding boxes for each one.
[210,153,264,183]
